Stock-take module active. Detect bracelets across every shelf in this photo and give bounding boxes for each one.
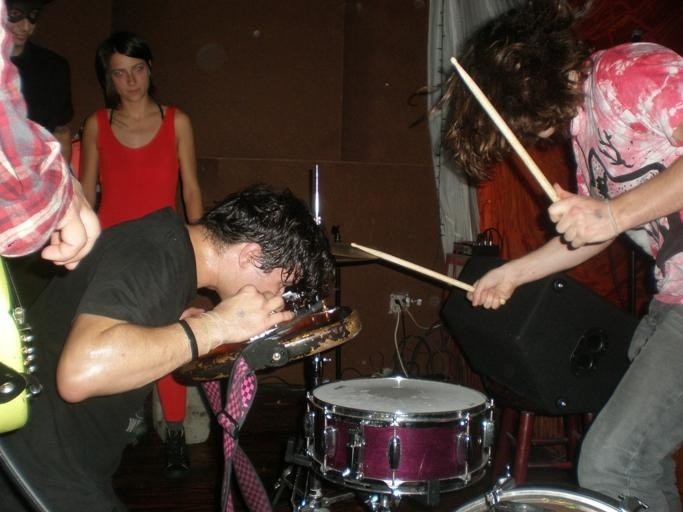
[179,319,198,362]
[605,198,617,234]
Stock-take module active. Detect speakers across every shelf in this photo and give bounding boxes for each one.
[439,253,651,417]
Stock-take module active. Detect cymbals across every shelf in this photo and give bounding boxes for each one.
[325,237,396,265]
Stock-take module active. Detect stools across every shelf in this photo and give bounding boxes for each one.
[493,406,598,489]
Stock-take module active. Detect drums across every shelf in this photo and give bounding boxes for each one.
[303,376,497,497]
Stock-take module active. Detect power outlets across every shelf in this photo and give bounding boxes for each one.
[389,294,408,313]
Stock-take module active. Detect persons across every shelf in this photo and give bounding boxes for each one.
[409,0,683,512]
[0,181,340,512]
[0,0,102,272]
[6,0,75,134]
[79,30,204,492]
